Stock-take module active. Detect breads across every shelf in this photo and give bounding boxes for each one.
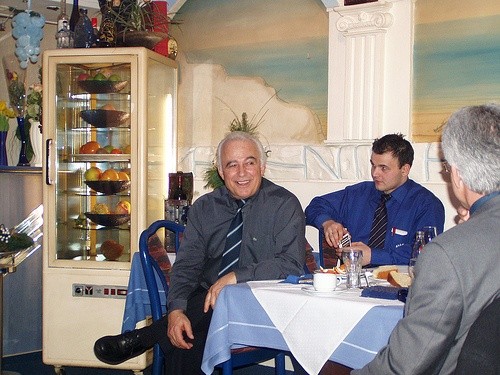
[387,271,411,287]
[373,266,398,279]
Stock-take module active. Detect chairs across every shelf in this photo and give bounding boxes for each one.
[139,220,285,375]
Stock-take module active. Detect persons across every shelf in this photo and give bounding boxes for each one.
[94,132,306,375]
[305,134,444,267]
[349,106,500,375]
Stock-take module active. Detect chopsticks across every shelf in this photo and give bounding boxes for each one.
[358,272,370,288]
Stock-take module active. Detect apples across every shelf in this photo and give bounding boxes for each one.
[100,103,116,111]
[115,200,131,214]
[85,167,130,181]
[78,72,121,81]
[78,141,123,154]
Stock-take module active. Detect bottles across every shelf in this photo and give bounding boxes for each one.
[57,0,94,49]
[412,231,426,258]
[426,231,433,244]
[92,17,99,35]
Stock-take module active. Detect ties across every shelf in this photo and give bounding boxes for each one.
[216,196,249,284]
[366,193,392,268]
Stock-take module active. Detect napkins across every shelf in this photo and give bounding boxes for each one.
[361,286,409,302]
[279,274,313,285]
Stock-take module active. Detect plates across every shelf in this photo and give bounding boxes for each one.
[302,286,346,294]
[71,154,130,162]
[314,269,348,278]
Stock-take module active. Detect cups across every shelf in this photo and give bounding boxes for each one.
[313,273,341,291]
[342,251,363,287]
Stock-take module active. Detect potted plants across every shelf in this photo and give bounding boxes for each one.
[95,0,186,50]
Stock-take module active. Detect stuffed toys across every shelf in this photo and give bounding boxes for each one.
[12,12,45,69]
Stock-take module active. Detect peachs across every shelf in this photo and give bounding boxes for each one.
[100,240,124,260]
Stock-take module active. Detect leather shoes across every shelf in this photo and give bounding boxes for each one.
[93,328,153,365]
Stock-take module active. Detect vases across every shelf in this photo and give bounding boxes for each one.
[17,117,36,166]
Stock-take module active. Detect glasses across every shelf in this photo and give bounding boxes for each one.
[441,159,458,173]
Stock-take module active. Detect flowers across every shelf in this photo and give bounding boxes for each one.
[3,55,42,122]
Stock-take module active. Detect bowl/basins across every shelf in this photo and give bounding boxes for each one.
[77,80,128,93]
[80,110,130,127]
[84,181,131,194]
[83,212,130,226]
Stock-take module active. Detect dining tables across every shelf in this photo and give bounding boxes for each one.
[201,267,406,375]
